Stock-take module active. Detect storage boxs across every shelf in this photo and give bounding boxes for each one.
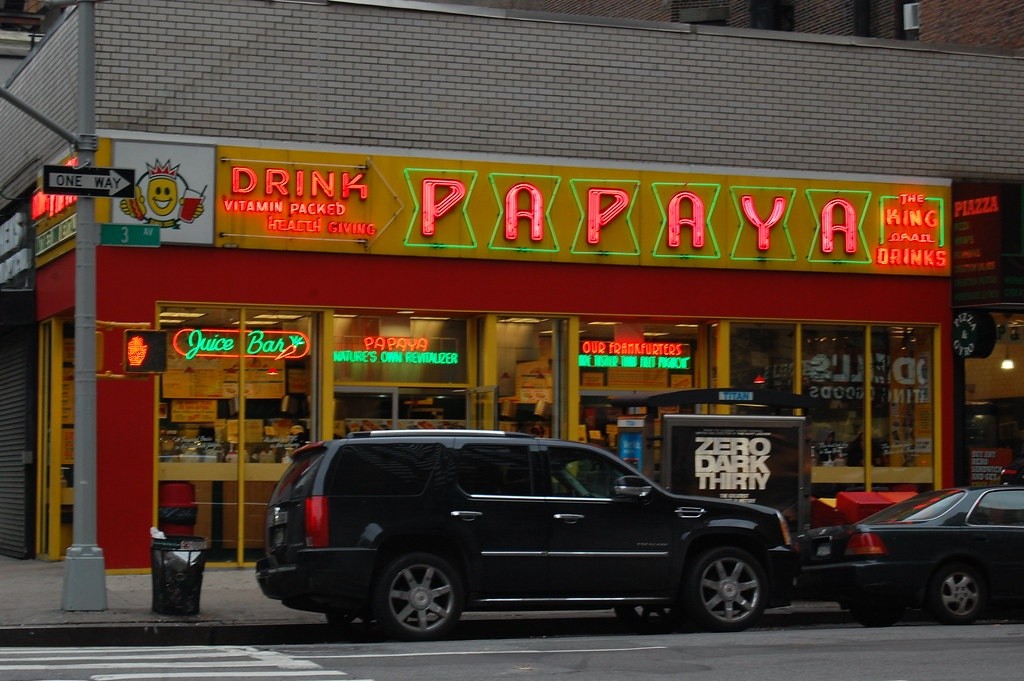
[153,317,312,442]
[498,328,554,435]
[579,365,691,462]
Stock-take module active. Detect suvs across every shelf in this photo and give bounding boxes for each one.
[254,430,796,638]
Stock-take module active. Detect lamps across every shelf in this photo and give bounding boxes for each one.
[1001,347,1015,370]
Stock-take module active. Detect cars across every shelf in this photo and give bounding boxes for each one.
[796,484,1024,628]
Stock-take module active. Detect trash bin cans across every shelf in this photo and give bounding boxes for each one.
[150,527,209,616]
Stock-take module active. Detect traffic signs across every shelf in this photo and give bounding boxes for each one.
[42,163,135,199]
